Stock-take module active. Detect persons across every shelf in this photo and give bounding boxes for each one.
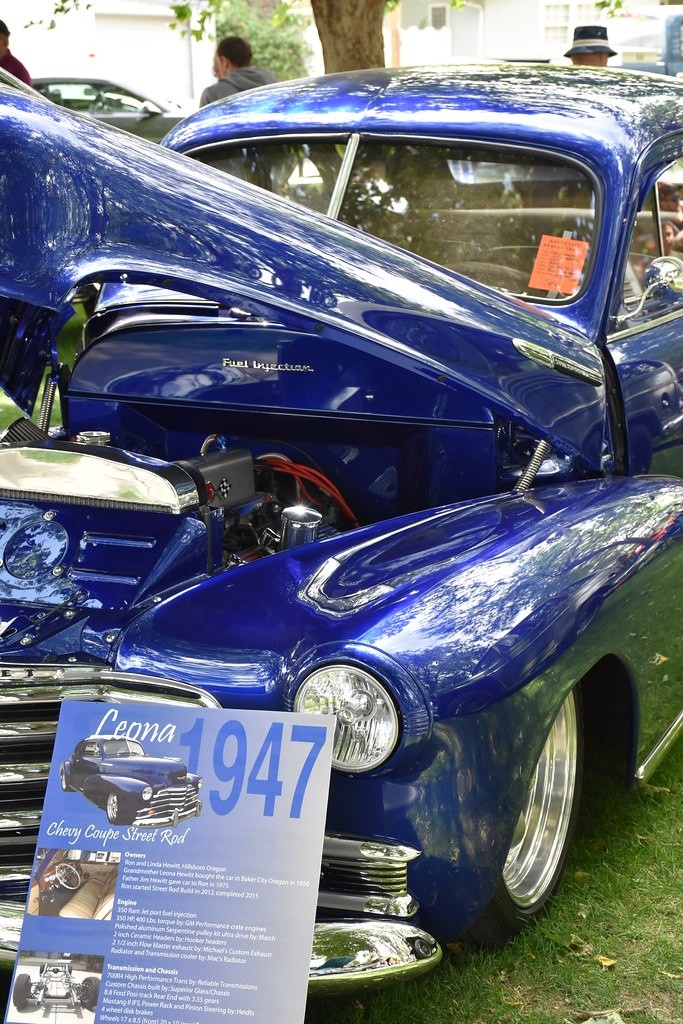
[564,26,617,67]
[0,19,33,86]
[658,183,683,261]
[199,37,278,108]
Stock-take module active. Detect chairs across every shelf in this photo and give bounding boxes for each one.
[441,261,547,298]
[479,244,540,275]
[51,89,62,105]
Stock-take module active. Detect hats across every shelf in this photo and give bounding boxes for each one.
[563,26,618,57]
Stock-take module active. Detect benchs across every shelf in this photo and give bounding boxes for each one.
[400,207,683,257]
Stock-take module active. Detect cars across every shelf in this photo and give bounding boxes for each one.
[0,64,683,1005]
[31,78,193,145]
